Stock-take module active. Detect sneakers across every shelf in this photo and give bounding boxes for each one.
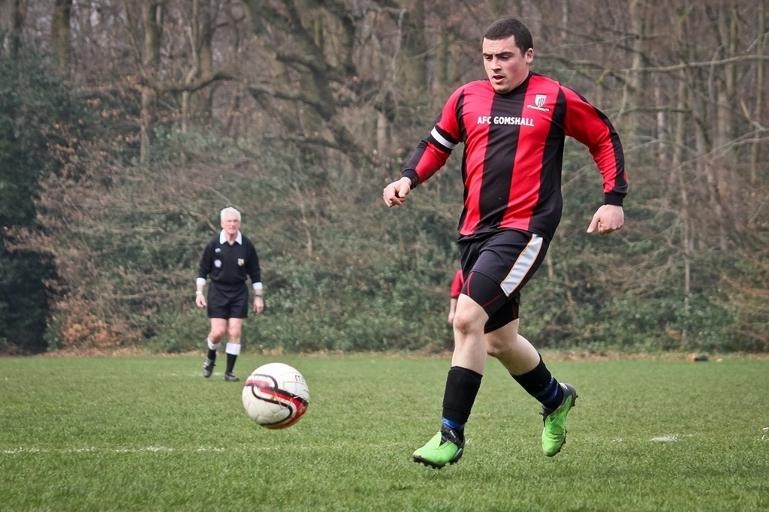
[224,372,239,382]
[411,429,465,470]
[202,356,216,378]
[539,380,579,457]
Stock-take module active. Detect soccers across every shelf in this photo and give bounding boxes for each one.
[241,363,309,429]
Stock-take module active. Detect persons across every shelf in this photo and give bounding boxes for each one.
[382,20,627,470]
[193,205,267,382]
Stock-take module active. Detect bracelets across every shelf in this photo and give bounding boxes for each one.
[255,294,264,298]
[194,290,204,295]
